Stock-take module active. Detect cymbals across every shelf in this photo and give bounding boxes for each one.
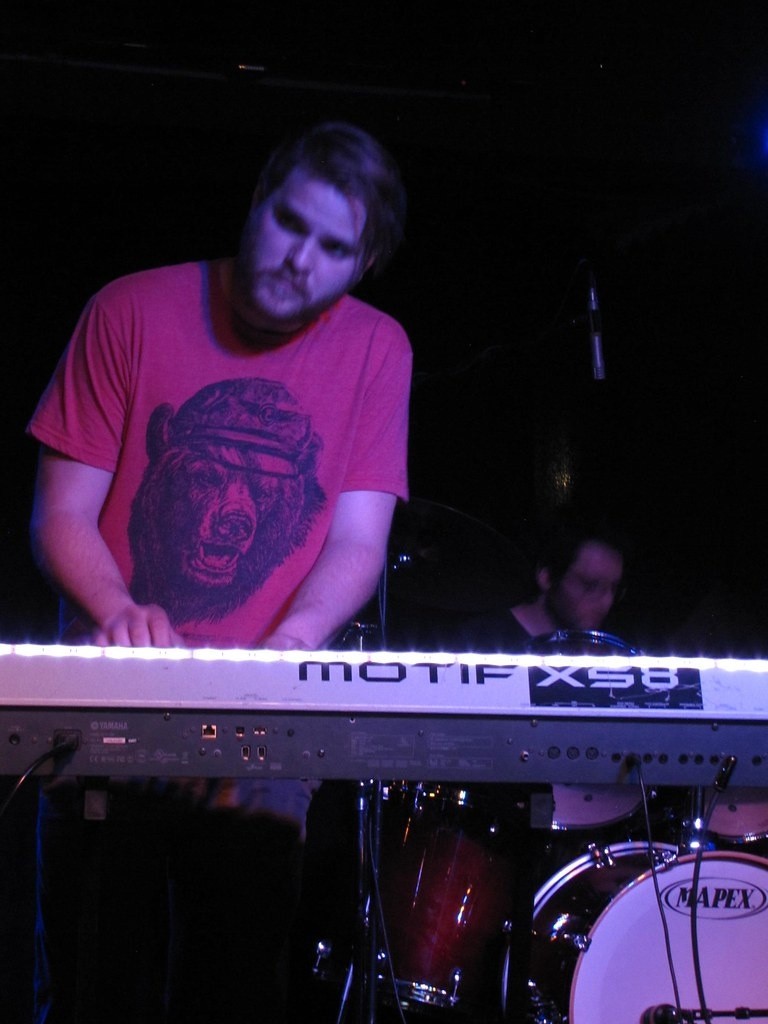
[380,493,534,614]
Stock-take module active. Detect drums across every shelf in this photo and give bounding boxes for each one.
[319,786,522,1021]
[497,839,767,1024]
[520,628,658,832]
[686,784,767,845]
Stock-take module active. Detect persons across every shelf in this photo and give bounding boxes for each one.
[443,534,624,653]
[25,123,414,844]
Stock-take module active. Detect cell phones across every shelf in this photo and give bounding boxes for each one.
[644,1004,679,1024]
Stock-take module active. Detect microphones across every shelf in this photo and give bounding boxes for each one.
[584,260,606,380]
